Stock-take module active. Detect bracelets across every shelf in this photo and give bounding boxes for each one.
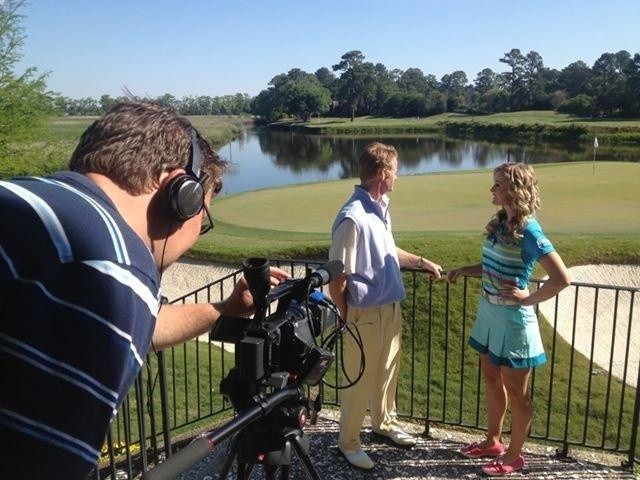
[418,256,424,269]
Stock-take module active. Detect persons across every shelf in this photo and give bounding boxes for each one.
[328,141,443,470]
[447,162,570,476]
[0,103,292,480]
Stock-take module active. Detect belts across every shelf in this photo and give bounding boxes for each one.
[481,290,518,306]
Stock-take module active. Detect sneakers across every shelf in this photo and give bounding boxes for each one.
[372,427,415,445]
[482,457,524,476]
[339,446,375,469]
[460,441,506,459]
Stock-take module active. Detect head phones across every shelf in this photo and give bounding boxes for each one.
[164,128,209,221]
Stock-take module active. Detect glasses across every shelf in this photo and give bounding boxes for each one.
[200,199,214,238]
